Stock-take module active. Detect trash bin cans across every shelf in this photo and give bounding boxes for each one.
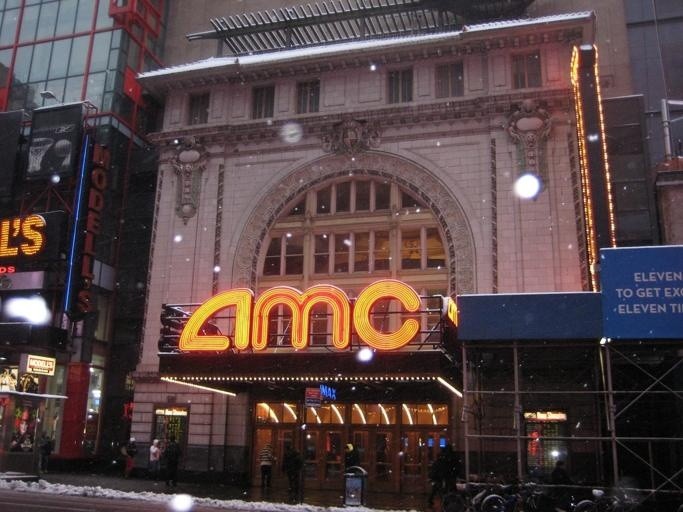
[343,466,367,507]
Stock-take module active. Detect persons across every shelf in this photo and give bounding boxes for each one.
[282,442,302,492]
[342,440,360,498]
[549,456,574,508]
[8,408,35,453]
[123,437,139,480]
[162,434,183,487]
[37,427,51,476]
[427,443,452,503]
[146,438,166,480]
[0,368,38,394]
[256,442,277,489]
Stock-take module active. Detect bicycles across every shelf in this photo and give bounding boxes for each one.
[439,472,637,511]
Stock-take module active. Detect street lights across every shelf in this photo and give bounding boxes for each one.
[597,337,613,433]
